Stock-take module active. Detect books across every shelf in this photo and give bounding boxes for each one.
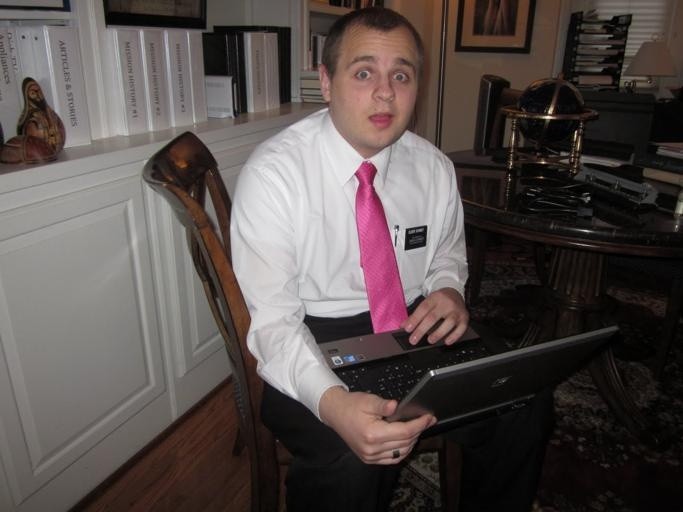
[301,1,388,105]
[641,143,683,186]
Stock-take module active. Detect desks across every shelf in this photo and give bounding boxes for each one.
[430,148,683,461]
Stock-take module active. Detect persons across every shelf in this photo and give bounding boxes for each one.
[229,9,562,511]
[17,73,66,157]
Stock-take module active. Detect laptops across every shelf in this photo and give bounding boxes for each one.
[316,317,618,444]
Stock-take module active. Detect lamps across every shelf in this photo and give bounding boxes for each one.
[622,35,679,97]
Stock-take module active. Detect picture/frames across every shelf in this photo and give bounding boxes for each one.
[452,1,539,56]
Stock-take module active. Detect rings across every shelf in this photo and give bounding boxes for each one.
[390,447,399,459]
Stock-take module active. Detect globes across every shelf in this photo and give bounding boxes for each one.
[500,73,600,203]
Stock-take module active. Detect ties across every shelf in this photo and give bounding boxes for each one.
[355,160,411,334]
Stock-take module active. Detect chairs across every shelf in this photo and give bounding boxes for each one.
[140,126,501,512]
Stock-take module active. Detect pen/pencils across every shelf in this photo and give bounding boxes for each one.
[393,224,399,247]
